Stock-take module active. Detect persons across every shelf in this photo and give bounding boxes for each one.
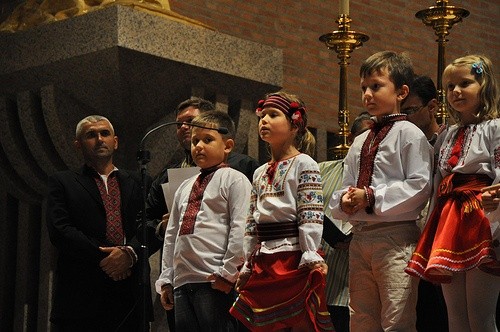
[47,115,161,332]
[405,55,500,332]
[136,97,261,332]
[400,74,450,332]
[228,91,330,332]
[156,110,253,332]
[329,51,434,332]
[306,112,376,332]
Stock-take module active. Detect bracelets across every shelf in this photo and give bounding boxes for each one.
[364,186,370,206]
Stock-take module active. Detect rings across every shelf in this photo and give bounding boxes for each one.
[489,208,491,212]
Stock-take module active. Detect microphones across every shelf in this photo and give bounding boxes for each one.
[139,122,228,149]
